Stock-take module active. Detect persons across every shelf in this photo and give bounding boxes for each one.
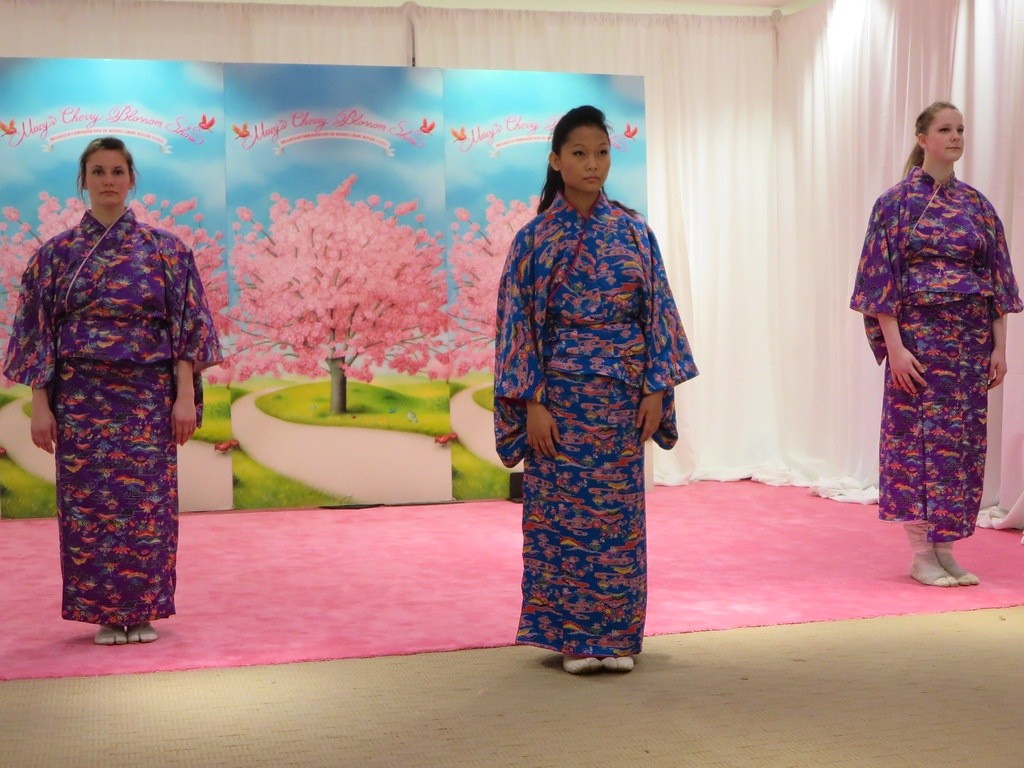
[3,138,224,644]
[849,100,1023,588]
[492,104,697,675]
